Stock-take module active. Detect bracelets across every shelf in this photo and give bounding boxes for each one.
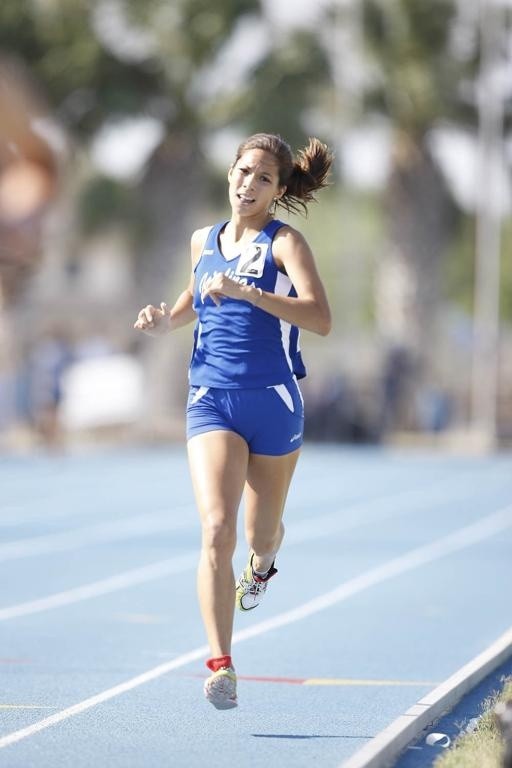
[253,287,263,306]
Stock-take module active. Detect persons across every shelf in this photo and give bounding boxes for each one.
[130,133,335,711]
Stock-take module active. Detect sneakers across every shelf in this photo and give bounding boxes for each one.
[235,549,277,611]
[204,655,237,709]
